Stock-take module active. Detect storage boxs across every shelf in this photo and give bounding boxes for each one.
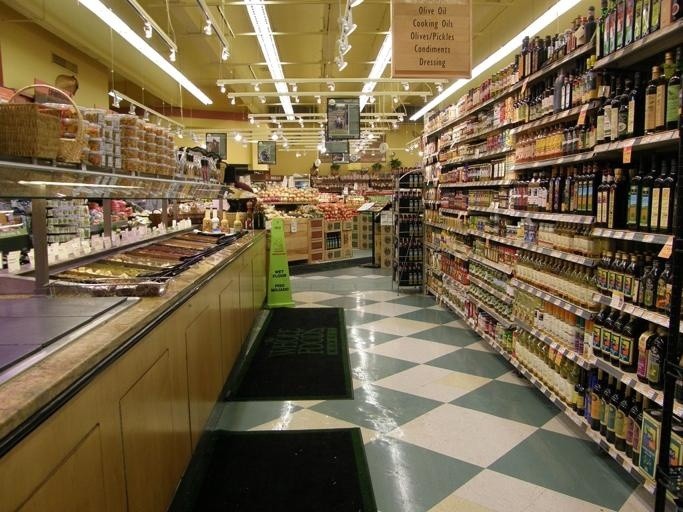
[311,211,395,268]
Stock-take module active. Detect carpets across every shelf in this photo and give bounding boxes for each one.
[167,427,378,512]
[222,307,354,402]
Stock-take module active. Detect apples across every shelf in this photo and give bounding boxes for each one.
[257,186,354,222]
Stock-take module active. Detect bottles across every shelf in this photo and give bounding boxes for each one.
[392,0,683,501]
[201,199,265,233]
[326,232,341,250]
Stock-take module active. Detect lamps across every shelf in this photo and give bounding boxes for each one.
[77,0,583,158]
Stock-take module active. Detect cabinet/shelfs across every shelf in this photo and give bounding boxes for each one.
[392,17,683,509]
[250,172,395,205]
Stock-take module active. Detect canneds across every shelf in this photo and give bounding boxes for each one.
[477,311,498,338]
[524,222,538,243]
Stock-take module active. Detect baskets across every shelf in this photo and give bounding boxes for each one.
[8,84,85,163]
[0,102,62,160]
